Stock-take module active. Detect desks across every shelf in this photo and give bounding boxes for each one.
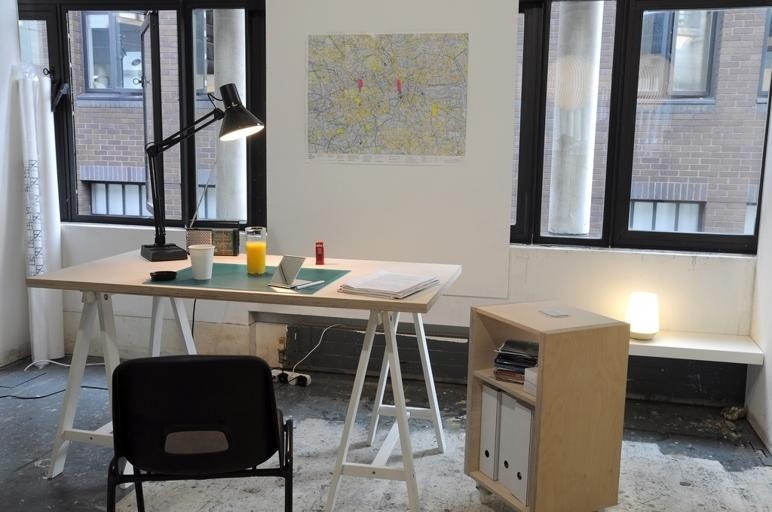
[26,248,463,512]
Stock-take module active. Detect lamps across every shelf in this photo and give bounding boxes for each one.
[141,83,265,262]
[626,292,660,340]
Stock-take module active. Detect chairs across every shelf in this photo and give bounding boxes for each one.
[107,355,293,512]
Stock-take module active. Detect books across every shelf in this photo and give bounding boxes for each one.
[494,338,539,384]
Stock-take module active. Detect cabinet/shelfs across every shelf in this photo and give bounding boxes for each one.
[464,301,630,512]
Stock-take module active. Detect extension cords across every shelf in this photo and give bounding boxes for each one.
[270,368,313,388]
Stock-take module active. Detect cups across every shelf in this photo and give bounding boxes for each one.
[188,243,215,281]
[245,225,267,276]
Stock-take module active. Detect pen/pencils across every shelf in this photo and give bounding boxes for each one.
[295,280,325,290]
